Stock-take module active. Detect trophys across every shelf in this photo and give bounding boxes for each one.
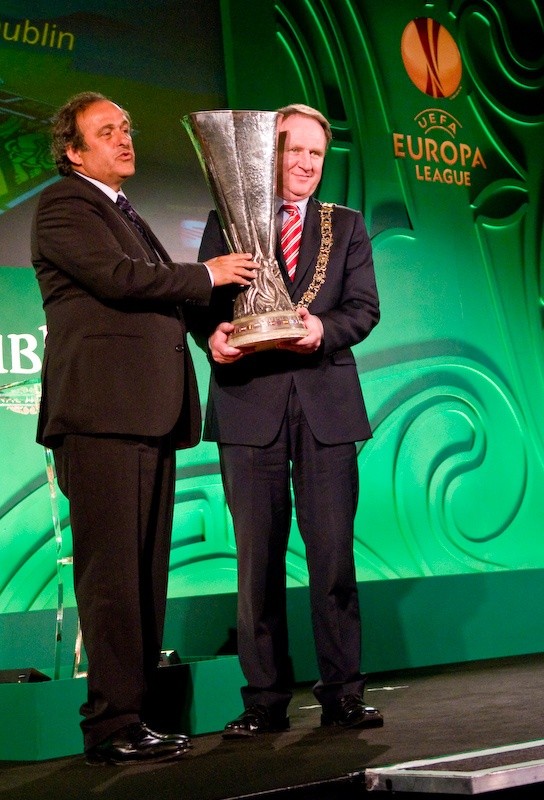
[180,109,308,351]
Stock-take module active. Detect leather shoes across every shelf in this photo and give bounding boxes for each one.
[221,704,291,738]
[84,721,193,766]
[320,697,383,730]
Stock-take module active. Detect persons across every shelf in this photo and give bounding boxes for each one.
[188,102,385,735]
[29,90,258,765]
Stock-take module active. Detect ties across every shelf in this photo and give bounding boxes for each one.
[116,193,162,265]
[279,203,303,283]
